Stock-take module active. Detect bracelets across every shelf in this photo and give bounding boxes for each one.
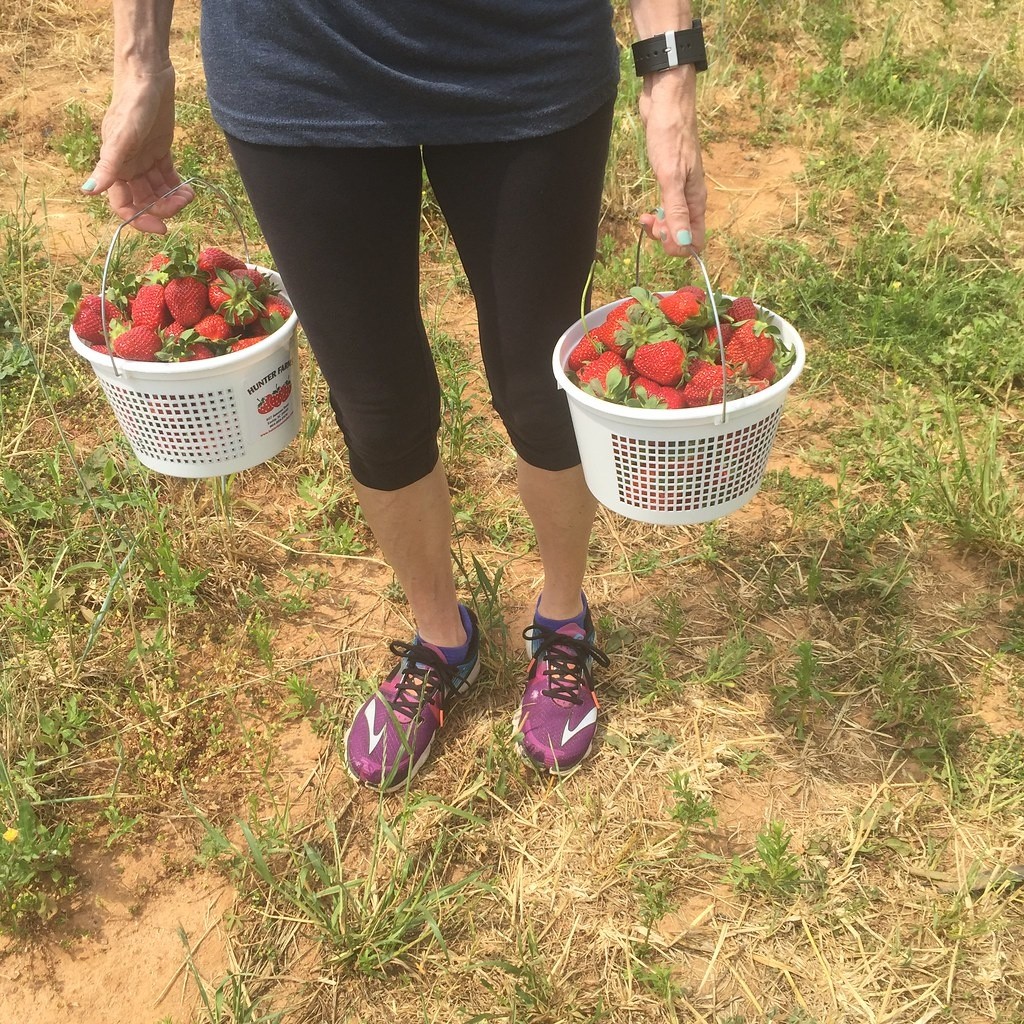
[631,19,707,76]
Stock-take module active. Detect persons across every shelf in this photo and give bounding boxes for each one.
[81,0,708,794]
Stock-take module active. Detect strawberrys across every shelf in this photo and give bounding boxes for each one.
[567,285,777,512]
[70,247,290,454]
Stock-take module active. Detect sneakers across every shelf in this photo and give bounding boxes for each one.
[344,606,478,795]
[517,591,600,774]
